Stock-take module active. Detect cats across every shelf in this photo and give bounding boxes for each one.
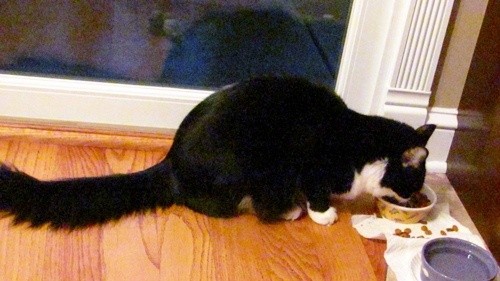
[0,77,437,234]
[1,6,347,90]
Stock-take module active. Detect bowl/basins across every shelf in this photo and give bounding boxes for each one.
[374,182,437,223]
[421,236,498,281]
